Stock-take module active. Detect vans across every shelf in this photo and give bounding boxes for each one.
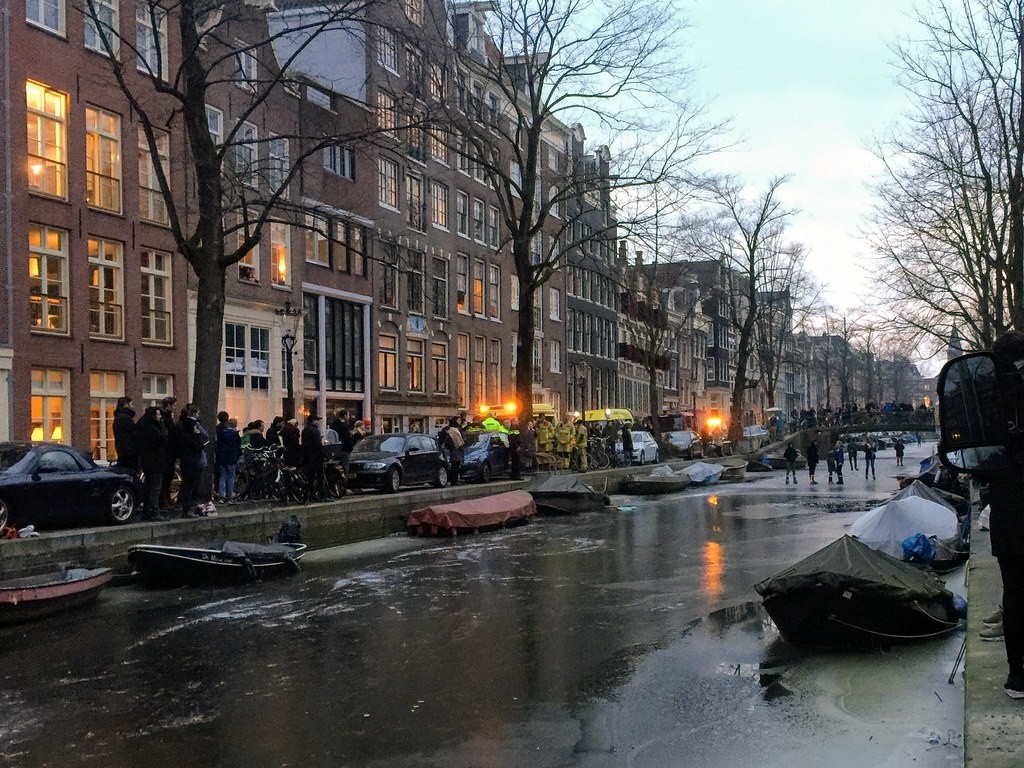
[479,404,559,427]
[572,408,634,433]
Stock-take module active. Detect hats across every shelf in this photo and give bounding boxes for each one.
[307,414,322,422]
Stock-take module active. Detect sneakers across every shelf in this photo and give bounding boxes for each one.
[981,608,1003,627]
[979,622,1005,641]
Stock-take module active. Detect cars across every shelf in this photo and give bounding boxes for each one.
[659,430,705,460]
[744,425,772,448]
[0,440,144,537]
[441,430,514,484]
[609,431,661,467]
[344,433,449,494]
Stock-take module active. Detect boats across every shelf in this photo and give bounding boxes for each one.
[0,567,113,623]
[753,534,968,651]
[126,515,308,591]
[620,449,808,495]
[847,452,970,573]
[405,489,538,537]
[527,475,611,515]
[841,432,918,451]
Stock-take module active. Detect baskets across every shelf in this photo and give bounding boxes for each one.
[244,447,266,465]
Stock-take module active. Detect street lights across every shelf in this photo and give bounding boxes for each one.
[689,377,700,432]
[574,357,588,426]
[275,297,302,422]
[759,392,766,426]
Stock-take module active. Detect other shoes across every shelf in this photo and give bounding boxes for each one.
[182,512,200,518]
[812,481,817,484]
[228,500,234,504]
[220,498,224,504]
[151,515,168,521]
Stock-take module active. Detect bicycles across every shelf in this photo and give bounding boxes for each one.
[138,442,347,507]
[571,433,632,473]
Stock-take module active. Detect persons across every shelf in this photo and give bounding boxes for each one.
[712,427,724,457]
[784,443,798,484]
[770,401,935,440]
[847,438,859,471]
[918,437,921,447]
[827,453,836,484]
[112,396,216,522]
[412,426,420,433]
[894,440,904,466]
[833,441,844,484]
[864,437,877,480]
[807,440,819,484]
[438,412,633,486]
[213,408,371,506]
[988,330,1024,700]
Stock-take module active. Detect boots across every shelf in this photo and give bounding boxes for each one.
[836,477,843,485]
